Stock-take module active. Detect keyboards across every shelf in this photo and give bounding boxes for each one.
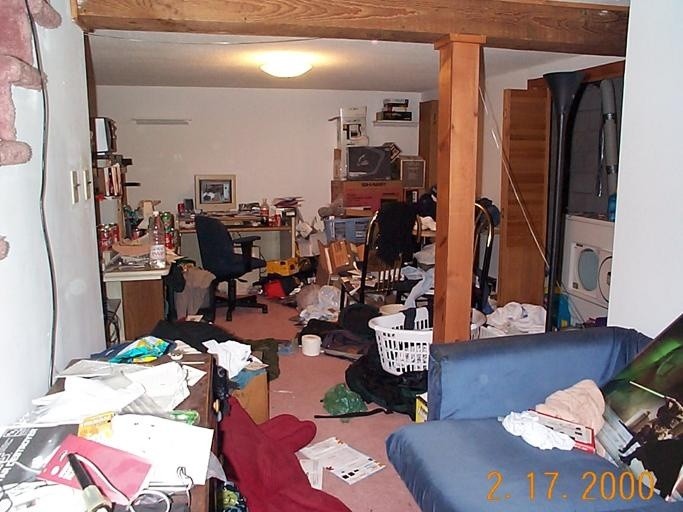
[220,220,244,225]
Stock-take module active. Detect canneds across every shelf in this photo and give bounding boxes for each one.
[159,211,179,251]
[178,203,185,213]
[275,214,281,227]
[97,223,119,259]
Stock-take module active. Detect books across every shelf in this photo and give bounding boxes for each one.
[526,408,596,455]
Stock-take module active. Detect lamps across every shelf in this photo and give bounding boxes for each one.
[259,43,313,78]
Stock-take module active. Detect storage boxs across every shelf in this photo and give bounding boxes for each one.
[232,351,269,424]
[331,180,403,210]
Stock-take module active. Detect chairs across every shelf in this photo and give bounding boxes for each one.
[339,207,421,310]
[396,202,494,310]
[195,216,268,321]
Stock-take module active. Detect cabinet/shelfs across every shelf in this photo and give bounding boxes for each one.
[39,353,220,512]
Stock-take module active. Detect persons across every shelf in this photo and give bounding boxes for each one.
[601,344,683,500]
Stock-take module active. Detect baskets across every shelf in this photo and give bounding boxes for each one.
[368,304,487,378]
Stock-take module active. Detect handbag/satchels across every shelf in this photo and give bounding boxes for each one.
[345,353,427,422]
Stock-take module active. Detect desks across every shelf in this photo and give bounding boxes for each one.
[178,217,296,259]
[103,260,173,341]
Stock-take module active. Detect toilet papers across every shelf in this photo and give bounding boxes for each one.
[302,335,322,356]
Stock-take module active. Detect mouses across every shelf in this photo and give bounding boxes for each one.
[252,222,258,227]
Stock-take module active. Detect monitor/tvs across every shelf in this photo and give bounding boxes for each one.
[195,174,237,216]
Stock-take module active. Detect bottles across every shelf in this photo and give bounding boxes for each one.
[259,198,269,227]
[148,211,166,269]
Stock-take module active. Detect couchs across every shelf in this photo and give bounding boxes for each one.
[385,327,683,512]
